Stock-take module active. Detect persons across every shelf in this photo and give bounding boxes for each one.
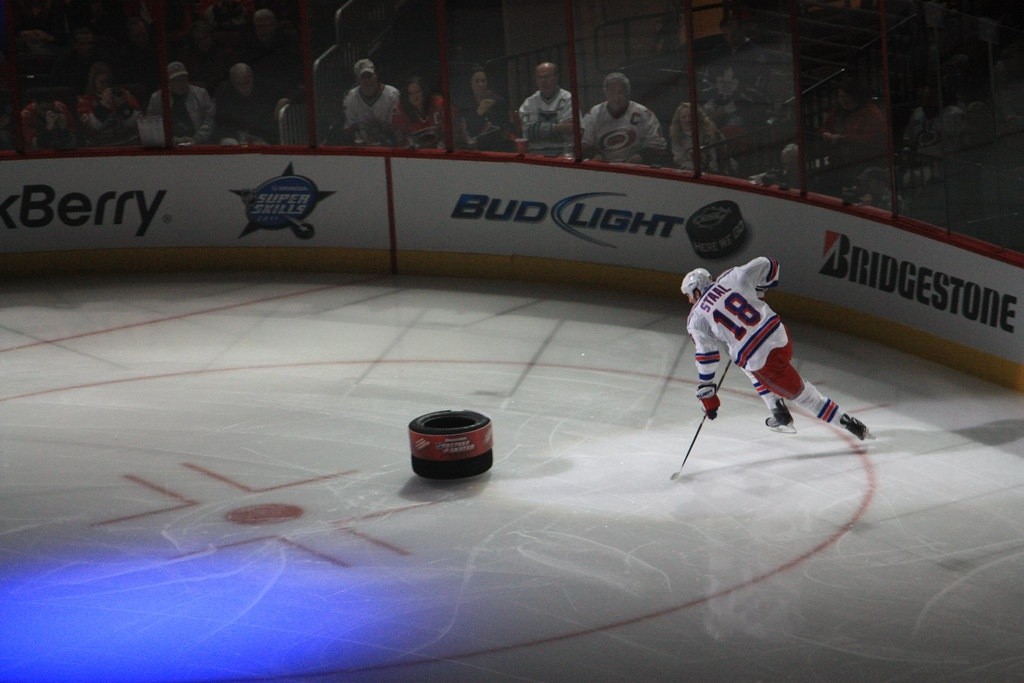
[389,77,459,151]
[2,0,297,144]
[341,59,406,149]
[516,61,585,159]
[455,67,513,150]
[897,43,981,162]
[755,141,828,200]
[816,65,890,206]
[670,94,764,180]
[680,257,860,438]
[581,70,669,166]
[702,15,773,104]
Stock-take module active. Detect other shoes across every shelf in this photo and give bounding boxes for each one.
[848,419,866,438]
[765,416,794,428]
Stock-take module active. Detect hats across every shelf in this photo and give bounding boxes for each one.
[167,63,187,79]
[353,60,376,77]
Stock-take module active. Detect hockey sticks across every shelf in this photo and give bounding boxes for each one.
[666,358,734,483]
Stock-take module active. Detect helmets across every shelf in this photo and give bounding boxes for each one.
[680,268,712,296]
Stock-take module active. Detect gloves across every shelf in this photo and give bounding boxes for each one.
[699,397,721,419]
